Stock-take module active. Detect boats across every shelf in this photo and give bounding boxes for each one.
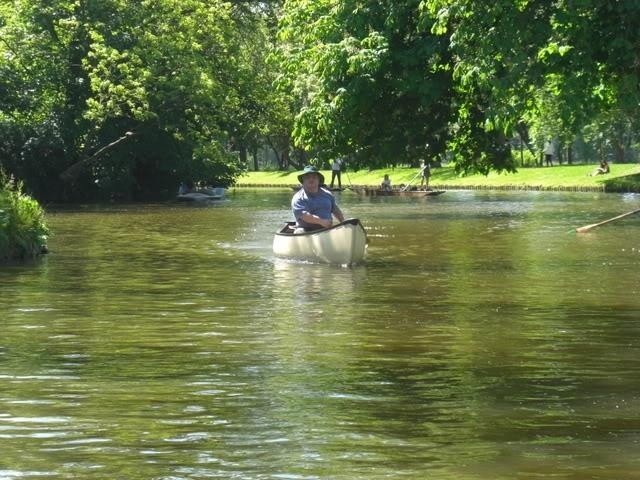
[272,218,367,267]
[176,187,224,201]
[348,186,446,197]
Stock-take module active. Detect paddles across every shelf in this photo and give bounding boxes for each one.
[403,166,428,190]
[576,210,640,232]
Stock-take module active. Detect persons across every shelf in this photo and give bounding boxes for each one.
[330,154,342,189]
[417,158,432,190]
[381,174,392,193]
[291,163,345,235]
[587,160,610,176]
[542,139,554,167]
[178,175,196,193]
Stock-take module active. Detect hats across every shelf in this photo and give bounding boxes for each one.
[295,164,325,186]
